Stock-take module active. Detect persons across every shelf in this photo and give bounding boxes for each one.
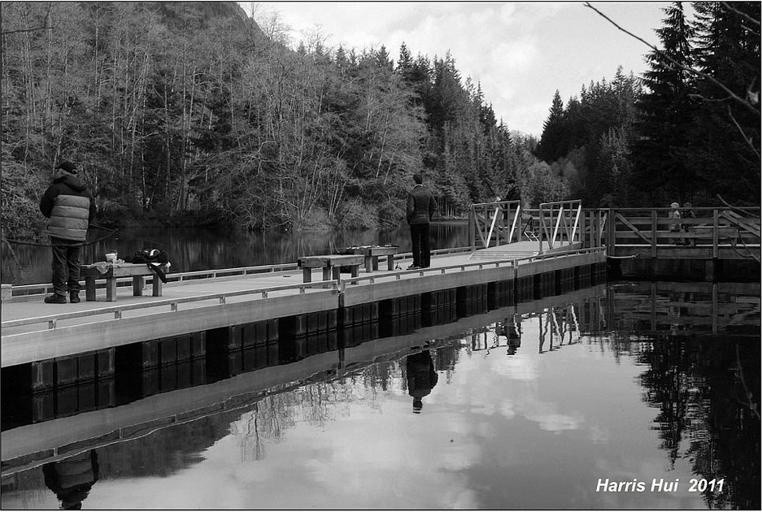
[38,160,97,303]
[668,202,680,244]
[406,349,438,409]
[405,174,437,270]
[42,449,99,510]
[503,318,520,355]
[496,178,520,220]
[680,202,696,245]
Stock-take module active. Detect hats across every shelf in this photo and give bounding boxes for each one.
[54,162,78,176]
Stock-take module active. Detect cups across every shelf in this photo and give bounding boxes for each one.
[105,253,117,265]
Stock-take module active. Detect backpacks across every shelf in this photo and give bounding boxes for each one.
[124,249,171,284]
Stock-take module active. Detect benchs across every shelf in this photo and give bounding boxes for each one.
[298,254,365,289]
[80,263,169,303]
[347,247,398,273]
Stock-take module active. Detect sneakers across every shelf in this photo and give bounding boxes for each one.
[70,293,81,304]
[44,293,67,304]
[406,263,428,270]
